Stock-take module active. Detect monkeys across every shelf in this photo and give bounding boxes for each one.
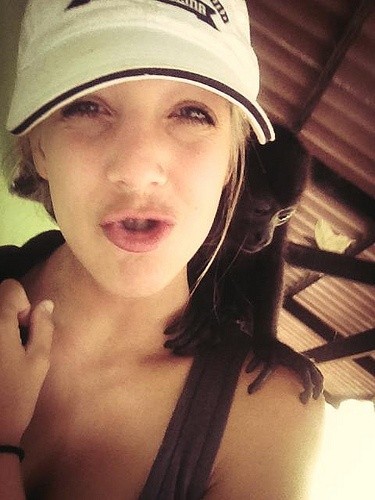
[0,120,326,405]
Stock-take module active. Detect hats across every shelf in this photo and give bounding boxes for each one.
[5,0,276,145]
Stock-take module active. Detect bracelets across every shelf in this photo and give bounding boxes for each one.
[0,445,24,463]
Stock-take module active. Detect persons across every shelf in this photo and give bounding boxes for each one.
[0,0,325,500]
